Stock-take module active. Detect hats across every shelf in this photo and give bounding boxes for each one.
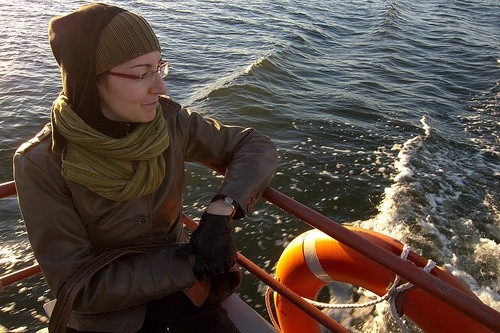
[93,10,162,76]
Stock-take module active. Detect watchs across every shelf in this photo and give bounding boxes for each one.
[223,195,238,218]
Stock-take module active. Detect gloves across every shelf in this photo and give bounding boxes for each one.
[175,211,241,280]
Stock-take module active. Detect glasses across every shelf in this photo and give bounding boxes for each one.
[103,62,169,84]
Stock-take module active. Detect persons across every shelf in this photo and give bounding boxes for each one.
[13,3,278,333]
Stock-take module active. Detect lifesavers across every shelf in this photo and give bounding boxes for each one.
[274,227,500,333]
